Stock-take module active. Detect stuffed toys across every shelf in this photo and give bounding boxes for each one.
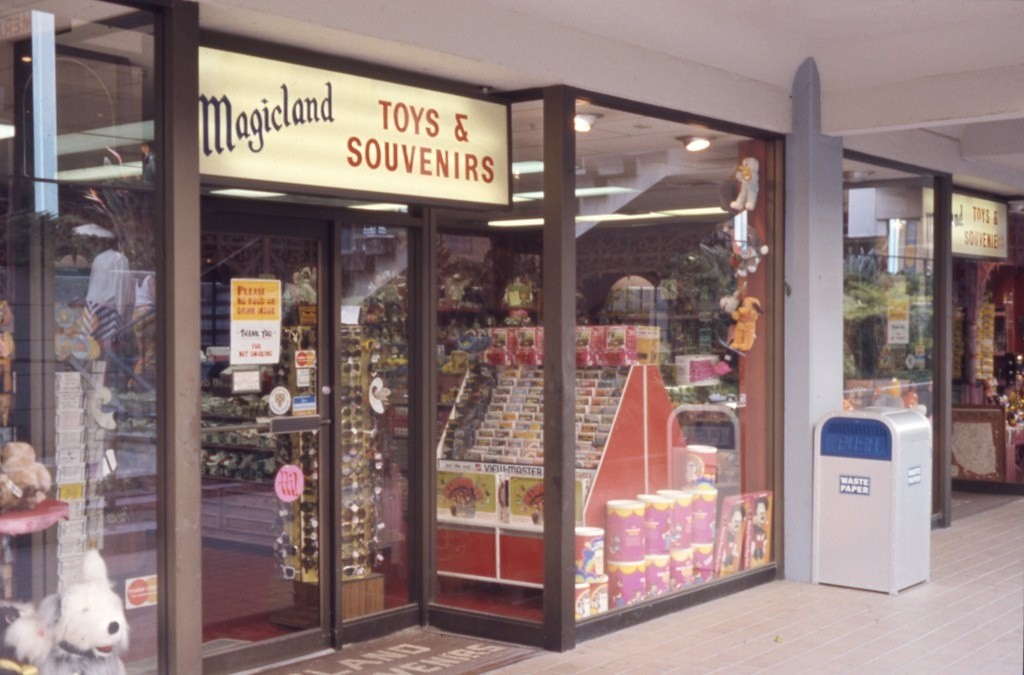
[0,443,51,511]
[731,157,758,211]
[732,223,769,278]
[6,550,130,675]
[730,298,763,351]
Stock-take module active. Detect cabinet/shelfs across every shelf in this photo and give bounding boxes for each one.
[994,311,1006,387]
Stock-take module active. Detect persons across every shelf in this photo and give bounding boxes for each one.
[448,273,465,308]
[383,276,404,321]
[285,272,317,324]
[79,230,130,377]
[843,275,870,378]
[896,271,913,294]
[128,256,156,388]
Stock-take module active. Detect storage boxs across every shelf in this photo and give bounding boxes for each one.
[508,474,590,528]
[436,469,507,523]
[741,489,772,569]
[713,493,751,579]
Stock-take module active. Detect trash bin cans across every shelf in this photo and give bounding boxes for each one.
[812,406,933,595]
[666,403,746,494]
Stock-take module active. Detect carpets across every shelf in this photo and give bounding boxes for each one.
[251,629,542,675]
